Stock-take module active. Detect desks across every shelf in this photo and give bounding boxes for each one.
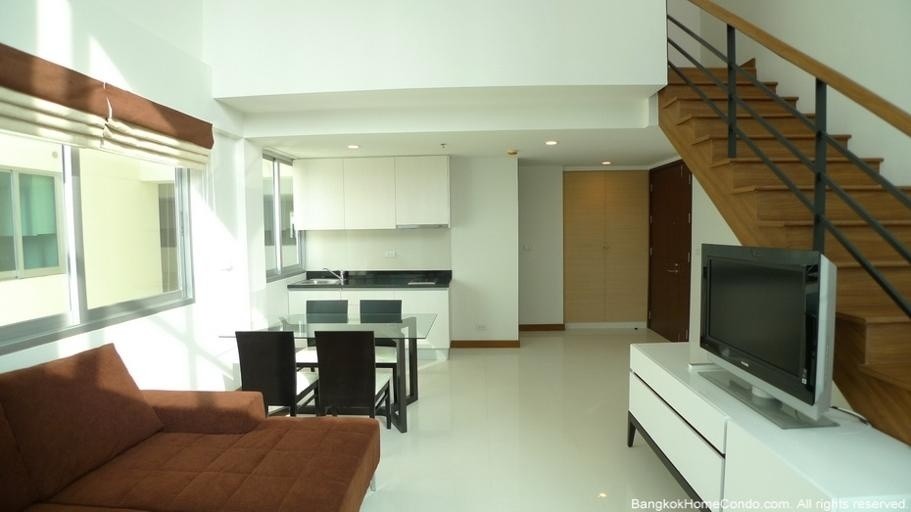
[216,312,439,434]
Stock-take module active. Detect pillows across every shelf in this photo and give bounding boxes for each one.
[0,342,167,503]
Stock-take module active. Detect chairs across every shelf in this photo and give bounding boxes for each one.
[304,299,350,349]
[358,299,404,350]
[314,328,395,430]
[234,328,320,417]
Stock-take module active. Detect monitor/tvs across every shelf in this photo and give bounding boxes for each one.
[697,244,839,429]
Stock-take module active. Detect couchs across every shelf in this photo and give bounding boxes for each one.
[0,387,383,512]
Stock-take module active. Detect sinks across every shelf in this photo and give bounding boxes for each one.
[294,279,341,285]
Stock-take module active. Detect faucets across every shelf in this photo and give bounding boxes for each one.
[323,267,344,286]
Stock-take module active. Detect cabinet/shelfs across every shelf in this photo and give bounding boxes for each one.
[626,342,910,512]
[291,157,451,229]
[404,289,449,348]
